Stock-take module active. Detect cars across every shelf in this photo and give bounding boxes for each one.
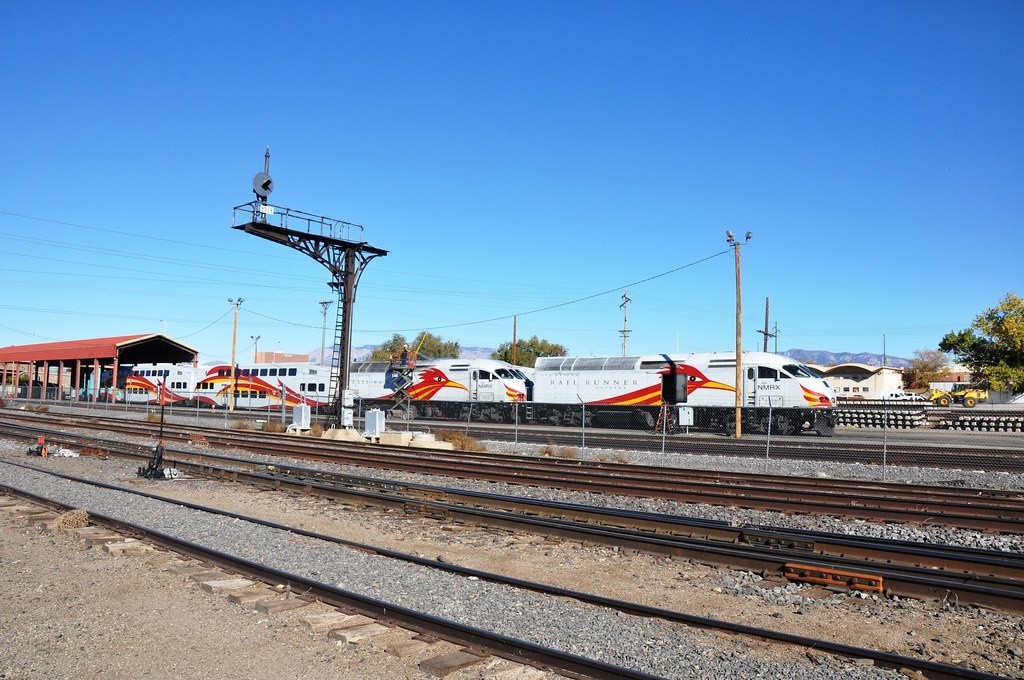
[888,388,926,402]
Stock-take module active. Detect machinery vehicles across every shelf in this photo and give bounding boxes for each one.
[929,382,988,408]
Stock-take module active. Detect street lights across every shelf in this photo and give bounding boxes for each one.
[227,297,244,412]
[318,300,334,364]
[726,230,753,439]
[249,335,261,364]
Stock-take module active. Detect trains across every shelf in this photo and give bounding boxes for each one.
[88,349,839,437]
